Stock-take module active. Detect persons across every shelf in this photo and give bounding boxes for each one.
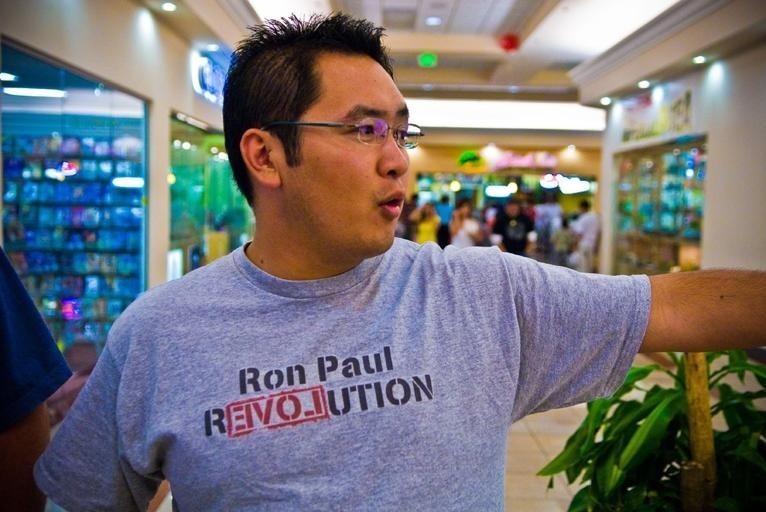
[31,11,766,511]
[0,247,73,512]
[393,189,601,274]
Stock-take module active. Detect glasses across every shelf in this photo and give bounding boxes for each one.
[259,116,425,151]
